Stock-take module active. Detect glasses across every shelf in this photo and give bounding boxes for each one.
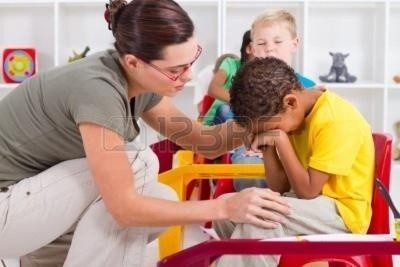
[137,44,203,81]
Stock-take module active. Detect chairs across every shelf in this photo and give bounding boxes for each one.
[276,132,395,267]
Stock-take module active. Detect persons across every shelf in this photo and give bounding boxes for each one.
[202,30,255,125]
[210,8,319,194]
[210,56,377,266]
[0,0,328,266]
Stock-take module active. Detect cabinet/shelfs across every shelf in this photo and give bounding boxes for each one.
[0,2,400,165]
